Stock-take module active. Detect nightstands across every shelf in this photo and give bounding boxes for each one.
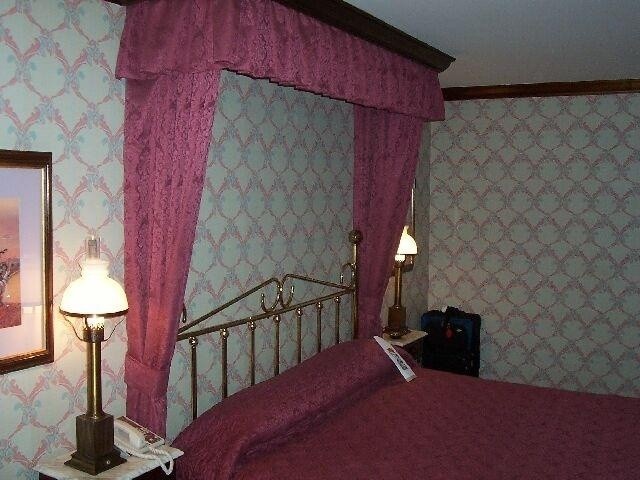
[32,441,184,480]
[377,328,428,370]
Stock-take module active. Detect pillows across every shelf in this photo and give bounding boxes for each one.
[167,338,421,480]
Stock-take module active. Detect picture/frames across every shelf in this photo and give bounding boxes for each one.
[0,149,54,375]
[374,178,415,278]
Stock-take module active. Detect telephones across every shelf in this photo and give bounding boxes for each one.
[114,416,165,453]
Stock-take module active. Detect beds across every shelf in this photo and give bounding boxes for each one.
[154,230,640,480]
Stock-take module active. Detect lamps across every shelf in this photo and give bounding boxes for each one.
[56,234,128,475]
[381,225,418,338]
